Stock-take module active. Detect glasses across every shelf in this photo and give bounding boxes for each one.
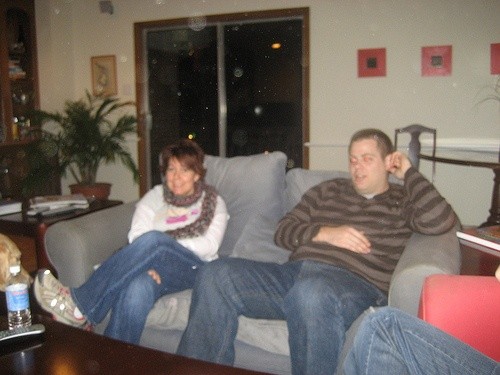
[349,154,385,167]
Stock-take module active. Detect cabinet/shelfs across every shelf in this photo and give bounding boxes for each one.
[0,0,42,145]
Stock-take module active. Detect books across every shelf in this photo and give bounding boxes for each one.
[8,60,27,80]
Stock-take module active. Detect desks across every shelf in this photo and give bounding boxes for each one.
[0,194,124,291]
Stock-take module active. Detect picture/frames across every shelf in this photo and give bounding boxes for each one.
[91,55,118,97]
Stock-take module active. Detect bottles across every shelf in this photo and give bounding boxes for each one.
[13,117,19,140]
[5,265,32,330]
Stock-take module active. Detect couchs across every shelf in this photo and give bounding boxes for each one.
[43,150,464,375]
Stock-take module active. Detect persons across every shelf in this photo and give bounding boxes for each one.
[33,141,230,345]
[332,306,500,375]
[176,128,457,375]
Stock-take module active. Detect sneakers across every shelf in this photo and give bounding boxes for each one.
[33,268,90,327]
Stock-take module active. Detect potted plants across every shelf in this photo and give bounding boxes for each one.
[16,90,145,207]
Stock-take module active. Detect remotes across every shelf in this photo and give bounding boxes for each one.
[0,324,46,349]
